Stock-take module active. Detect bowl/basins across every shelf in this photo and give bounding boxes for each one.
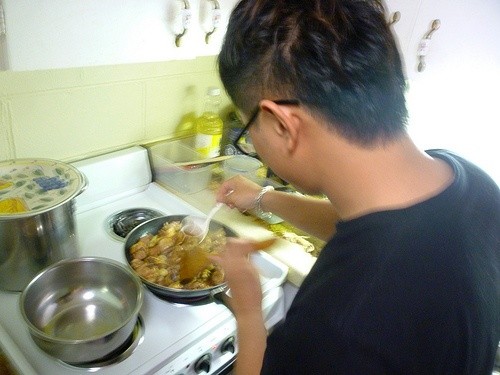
[17,256,143,363]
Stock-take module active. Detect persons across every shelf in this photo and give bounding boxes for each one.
[217,0,500,375]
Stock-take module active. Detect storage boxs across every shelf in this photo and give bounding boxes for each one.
[149,142,214,195]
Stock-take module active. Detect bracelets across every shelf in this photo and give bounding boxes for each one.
[256,185,274,212]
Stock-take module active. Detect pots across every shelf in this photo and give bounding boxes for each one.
[0,158,88,292]
[123,215,270,339]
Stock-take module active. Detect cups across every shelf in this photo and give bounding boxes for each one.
[224,157,263,183]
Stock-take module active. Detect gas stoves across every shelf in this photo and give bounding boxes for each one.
[0,148,287,375]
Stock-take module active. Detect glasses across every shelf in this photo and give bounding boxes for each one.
[232,98,300,159]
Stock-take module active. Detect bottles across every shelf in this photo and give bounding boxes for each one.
[192,89,224,160]
